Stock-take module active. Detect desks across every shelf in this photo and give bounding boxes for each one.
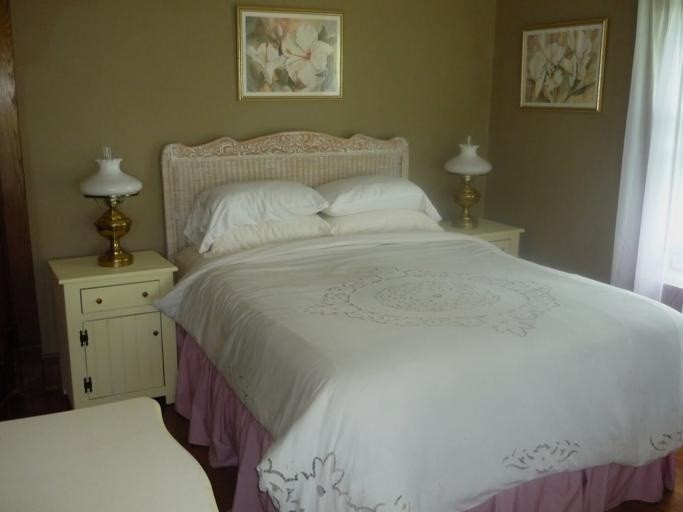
[0,397,219,512]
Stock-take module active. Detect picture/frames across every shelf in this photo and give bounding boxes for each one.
[517,16,609,113]
[234,3,346,102]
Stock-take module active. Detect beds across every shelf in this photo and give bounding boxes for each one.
[156,130,682,512]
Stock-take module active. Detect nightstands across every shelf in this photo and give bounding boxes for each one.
[47,250,179,411]
[439,216,525,259]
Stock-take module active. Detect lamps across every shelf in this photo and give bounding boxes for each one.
[441,136,494,232]
[78,147,143,268]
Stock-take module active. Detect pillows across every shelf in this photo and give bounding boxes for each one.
[182,173,444,260]
